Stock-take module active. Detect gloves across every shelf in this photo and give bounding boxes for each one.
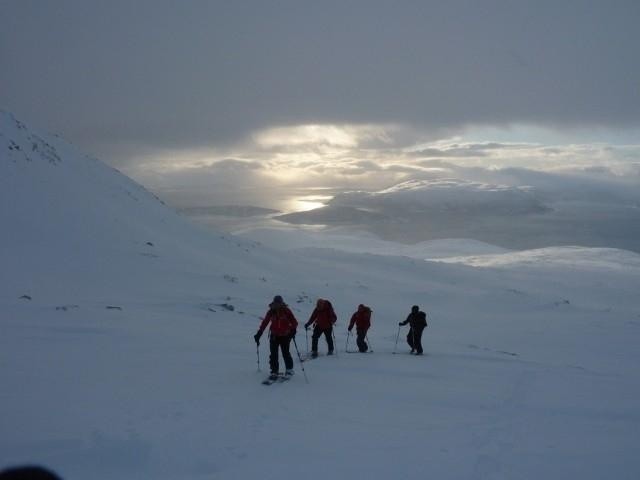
[305,324,308,328]
[255,330,263,342]
[289,328,296,338]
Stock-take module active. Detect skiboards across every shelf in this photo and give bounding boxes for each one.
[393,350,425,355]
[261,370,295,385]
[345,350,372,353]
[300,350,334,362]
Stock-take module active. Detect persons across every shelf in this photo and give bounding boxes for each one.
[304,298,339,360]
[252,294,299,381]
[349,302,373,355]
[398,304,429,355]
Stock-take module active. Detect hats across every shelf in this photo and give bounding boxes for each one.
[274,296,283,303]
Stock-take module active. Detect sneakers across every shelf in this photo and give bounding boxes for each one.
[328,350,332,355]
[285,369,294,376]
[270,373,278,379]
[311,351,317,356]
